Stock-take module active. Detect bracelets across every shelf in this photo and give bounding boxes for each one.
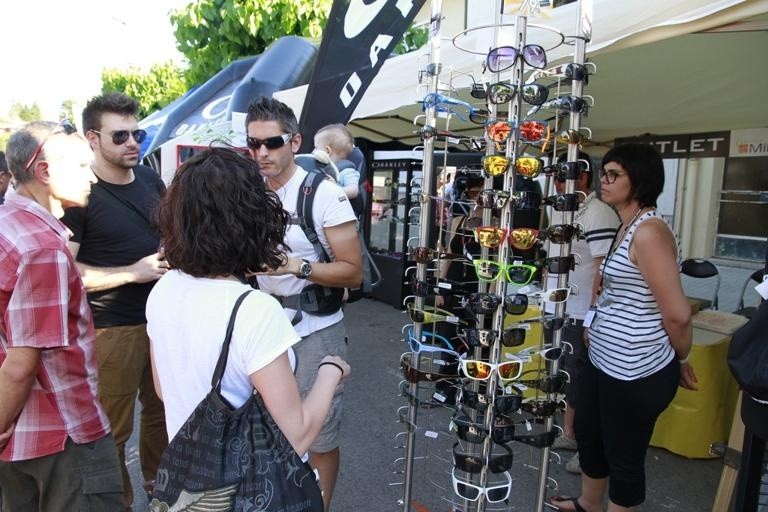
[317,361,344,375]
[679,354,691,364]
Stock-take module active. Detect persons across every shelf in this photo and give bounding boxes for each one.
[549,143,699,512]
[541,151,621,475]
[59,93,170,512]
[421,177,483,409]
[311,122,360,198]
[245,96,363,512]
[146,148,351,512]
[0,121,126,512]
[0,151,12,206]
[436,168,449,253]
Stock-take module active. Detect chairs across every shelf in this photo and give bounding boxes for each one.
[679,258,720,310]
[732,268,765,319]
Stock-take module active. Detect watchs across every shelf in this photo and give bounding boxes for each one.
[296,258,313,279]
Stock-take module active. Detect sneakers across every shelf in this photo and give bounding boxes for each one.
[565,450,582,474]
[550,435,577,450]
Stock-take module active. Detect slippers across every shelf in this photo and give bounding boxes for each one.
[550,494,586,512]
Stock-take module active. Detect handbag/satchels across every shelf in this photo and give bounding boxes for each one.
[147,289,326,511]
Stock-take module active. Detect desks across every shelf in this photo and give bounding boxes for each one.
[649,310,746,459]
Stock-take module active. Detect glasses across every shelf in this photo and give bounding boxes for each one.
[417,62,477,96]
[545,224,582,244]
[513,418,559,448]
[504,342,567,362]
[485,81,549,114]
[516,313,571,331]
[463,328,526,348]
[26,118,80,172]
[471,260,537,287]
[527,285,574,304]
[478,188,541,212]
[452,414,516,443]
[540,193,580,212]
[471,83,490,99]
[482,44,548,75]
[406,245,473,265]
[469,108,508,124]
[451,441,514,474]
[458,352,523,381]
[422,92,474,122]
[541,253,576,274]
[419,125,481,152]
[89,127,148,145]
[482,154,545,177]
[540,161,581,180]
[525,62,589,89]
[520,400,565,417]
[522,96,589,122]
[246,133,294,151]
[464,190,481,199]
[411,272,470,299]
[469,292,528,315]
[407,302,469,327]
[451,466,514,503]
[470,135,507,153]
[461,380,524,414]
[483,119,551,152]
[599,168,627,184]
[550,128,581,145]
[400,354,460,384]
[476,225,539,250]
[402,383,459,412]
[504,369,566,394]
[407,328,460,358]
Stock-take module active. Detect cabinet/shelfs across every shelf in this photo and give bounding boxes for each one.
[387,0,597,512]
